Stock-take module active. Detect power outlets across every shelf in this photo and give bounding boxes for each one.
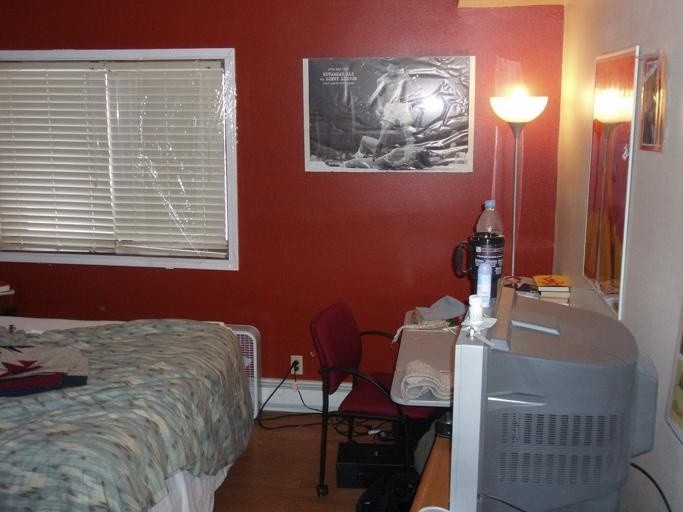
[290,355,304,376]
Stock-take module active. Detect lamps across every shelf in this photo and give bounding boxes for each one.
[489,84,548,277]
[592,87,632,288]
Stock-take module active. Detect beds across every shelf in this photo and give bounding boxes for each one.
[0,317,254,512]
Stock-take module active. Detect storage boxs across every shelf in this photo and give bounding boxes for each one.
[335,442,415,489]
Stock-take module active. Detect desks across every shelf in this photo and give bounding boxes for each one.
[406,434,452,512]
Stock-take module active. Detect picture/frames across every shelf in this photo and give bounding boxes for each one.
[664,330,683,446]
[639,51,666,153]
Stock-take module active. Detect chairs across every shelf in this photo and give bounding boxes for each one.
[308,302,446,498]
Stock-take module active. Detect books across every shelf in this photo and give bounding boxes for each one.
[497,274,572,304]
[0,278,9,293]
[590,276,620,303]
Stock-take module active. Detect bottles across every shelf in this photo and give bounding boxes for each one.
[476,200,503,237]
[476,263,492,307]
[467,294,483,322]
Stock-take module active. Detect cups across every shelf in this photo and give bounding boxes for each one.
[454,232,504,280]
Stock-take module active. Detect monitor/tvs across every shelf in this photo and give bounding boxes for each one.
[449,286,659,512]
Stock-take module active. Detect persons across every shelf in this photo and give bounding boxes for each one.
[322,134,437,171]
[365,59,418,160]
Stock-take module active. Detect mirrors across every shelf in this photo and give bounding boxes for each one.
[582,45,638,323]
